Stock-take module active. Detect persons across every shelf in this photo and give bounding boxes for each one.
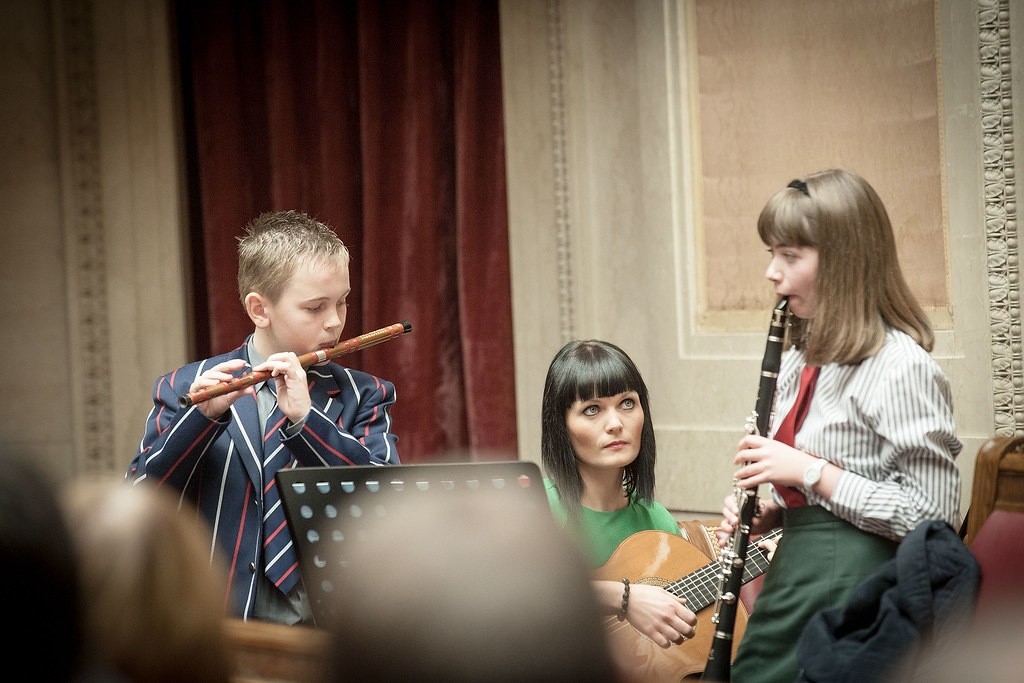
[540,338,787,654]
[120,212,404,626]
[716,166,964,683]
[1,460,78,683]
[317,463,619,683]
[54,468,238,683]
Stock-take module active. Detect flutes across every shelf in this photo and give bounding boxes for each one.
[702,296,792,683]
[177,319,413,409]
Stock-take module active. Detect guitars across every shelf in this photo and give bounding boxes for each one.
[587,524,786,683]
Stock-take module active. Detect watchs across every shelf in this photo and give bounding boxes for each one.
[803,457,828,492]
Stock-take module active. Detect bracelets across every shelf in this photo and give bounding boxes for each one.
[616,578,629,622]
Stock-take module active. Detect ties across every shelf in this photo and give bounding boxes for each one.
[771,347,829,507]
[264,379,302,596]
